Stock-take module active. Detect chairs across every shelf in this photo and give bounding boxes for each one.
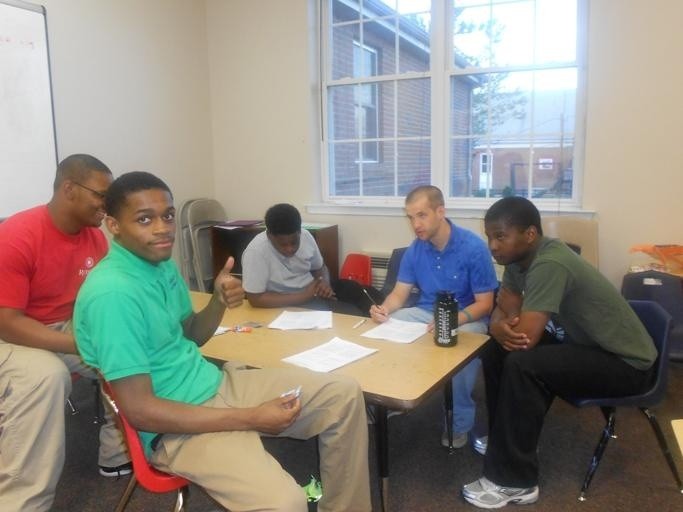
[189,199,225,292]
[95,368,192,512]
[621,270,683,366]
[339,253,371,288]
[577,300,683,503]
[68,371,81,415]
[380,248,421,308]
[178,198,197,290]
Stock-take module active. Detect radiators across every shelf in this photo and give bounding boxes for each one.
[365,250,419,293]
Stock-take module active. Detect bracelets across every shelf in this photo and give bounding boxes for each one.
[460,310,474,323]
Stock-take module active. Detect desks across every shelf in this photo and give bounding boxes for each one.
[184,291,491,511]
[628,245,683,277]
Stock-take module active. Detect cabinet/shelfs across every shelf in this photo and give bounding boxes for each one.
[210,219,338,291]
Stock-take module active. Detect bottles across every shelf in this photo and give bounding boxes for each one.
[433,291,459,347]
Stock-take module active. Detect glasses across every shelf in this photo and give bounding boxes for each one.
[69,180,105,199]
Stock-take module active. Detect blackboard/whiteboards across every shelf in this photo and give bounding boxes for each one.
[0,0,58,223]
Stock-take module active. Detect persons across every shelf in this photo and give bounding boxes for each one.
[241,203,364,316]
[365,185,499,447]
[462,198,660,508]
[73,172,371,512]
[1,154,133,512]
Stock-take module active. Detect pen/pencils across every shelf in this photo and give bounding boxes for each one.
[363,289,387,317]
[352,318,367,331]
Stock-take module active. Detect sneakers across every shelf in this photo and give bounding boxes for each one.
[463,476,539,509]
[441,430,468,448]
[474,436,488,455]
[365,406,403,424]
[97,461,134,477]
